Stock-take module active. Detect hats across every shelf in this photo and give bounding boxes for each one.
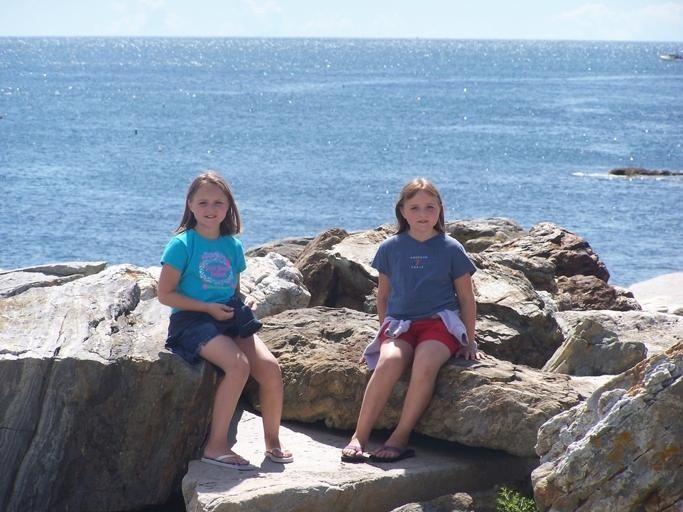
[216,299,263,338]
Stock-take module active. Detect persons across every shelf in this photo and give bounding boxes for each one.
[158,171,294,470]
[338,176,486,462]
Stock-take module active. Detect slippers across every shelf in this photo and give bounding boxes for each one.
[368,446,414,462]
[264,447,294,463]
[341,444,364,462]
[200,452,255,471]
